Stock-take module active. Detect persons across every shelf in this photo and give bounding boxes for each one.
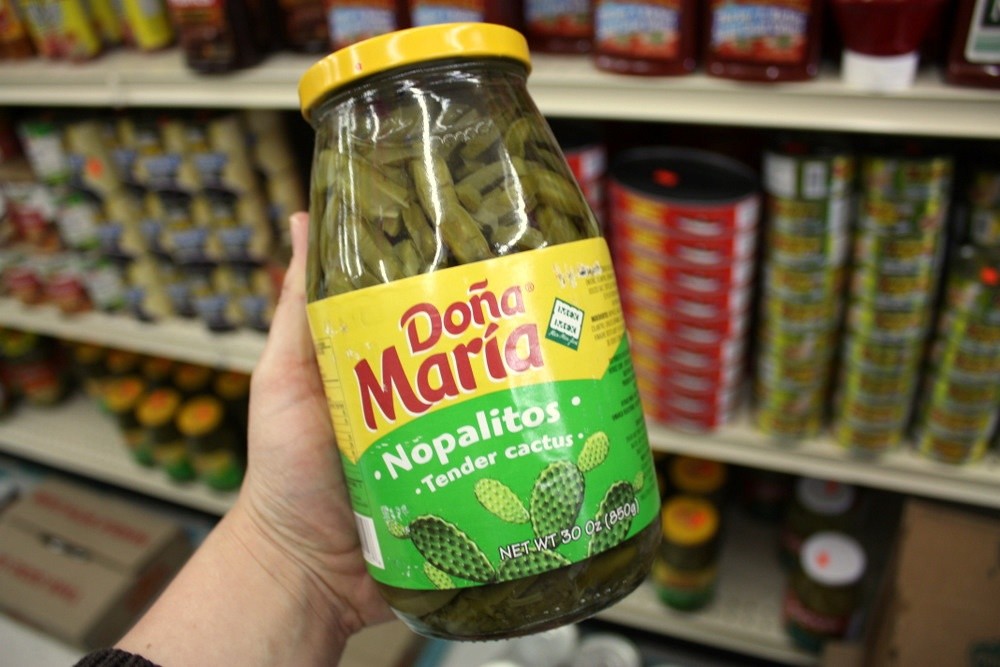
[72,211,403,667]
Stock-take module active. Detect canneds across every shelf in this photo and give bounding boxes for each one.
[0,0,1000,655]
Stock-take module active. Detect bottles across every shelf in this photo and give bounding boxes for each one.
[832,0,951,90]
[299,22,662,641]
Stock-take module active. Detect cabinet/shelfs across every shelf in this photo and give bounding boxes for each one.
[0,46,1000,667]
